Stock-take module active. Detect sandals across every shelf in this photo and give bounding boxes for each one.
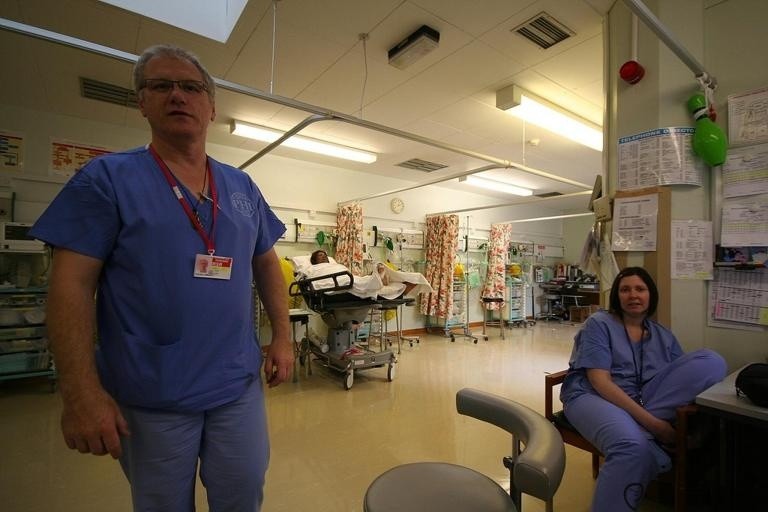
[648,440,673,473]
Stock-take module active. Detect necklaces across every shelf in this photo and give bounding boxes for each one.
[163,161,207,229]
[623,323,645,407]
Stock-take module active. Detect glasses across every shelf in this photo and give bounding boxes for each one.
[148,79,207,95]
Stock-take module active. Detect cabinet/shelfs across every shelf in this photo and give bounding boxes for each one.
[0,286,58,394]
[426,272,470,337]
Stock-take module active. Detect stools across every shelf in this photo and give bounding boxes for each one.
[386,298,420,347]
[481,297,505,341]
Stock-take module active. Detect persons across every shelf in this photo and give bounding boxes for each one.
[28,45,295,512]
[310,250,421,296]
[559,267,727,512]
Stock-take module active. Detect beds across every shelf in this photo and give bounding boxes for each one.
[288,254,415,390]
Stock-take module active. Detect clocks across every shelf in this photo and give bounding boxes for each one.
[390,198,404,214]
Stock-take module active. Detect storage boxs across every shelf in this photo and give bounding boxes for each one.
[570,307,589,322]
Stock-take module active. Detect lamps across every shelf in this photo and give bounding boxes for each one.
[497,85,604,155]
[458,174,534,196]
[229,118,377,164]
[388,24,440,71]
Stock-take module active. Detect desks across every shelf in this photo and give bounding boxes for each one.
[695,362,767,424]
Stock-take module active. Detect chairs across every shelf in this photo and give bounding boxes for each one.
[366,387,567,509]
[536,283,563,323]
[545,369,713,511]
